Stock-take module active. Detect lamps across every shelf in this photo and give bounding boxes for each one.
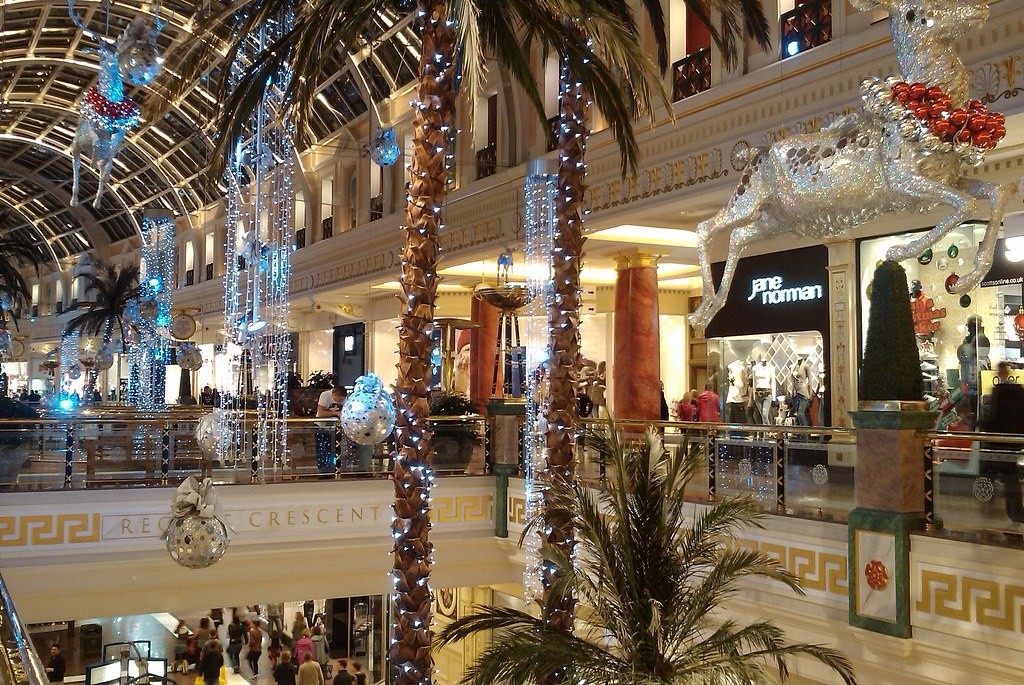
[225,338,245,357]
[1004,235,1023,263]
[146,277,158,291]
[784,26,806,53]
[240,311,270,331]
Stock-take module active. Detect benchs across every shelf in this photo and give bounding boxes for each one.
[85,433,390,486]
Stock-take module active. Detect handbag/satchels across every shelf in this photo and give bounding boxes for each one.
[326,662,333,680]
[187,639,195,656]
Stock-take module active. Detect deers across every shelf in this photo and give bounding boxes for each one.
[68,1,175,208]
[687,0,1008,332]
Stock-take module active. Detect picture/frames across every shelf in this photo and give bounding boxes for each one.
[433,589,460,625]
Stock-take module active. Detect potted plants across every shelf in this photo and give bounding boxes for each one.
[426,388,485,473]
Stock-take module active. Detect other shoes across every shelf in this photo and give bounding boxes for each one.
[245,639,248,644]
[251,674,261,680]
[234,668,239,673]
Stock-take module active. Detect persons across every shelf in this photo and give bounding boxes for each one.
[963,314,991,432]
[8,387,127,406]
[575,387,594,452]
[529,368,548,442]
[385,400,396,480]
[313,384,347,480]
[170,600,367,685]
[658,379,723,453]
[909,279,939,391]
[723,340,826,444]
[44,644,65,685]
[199,386,272,410]
